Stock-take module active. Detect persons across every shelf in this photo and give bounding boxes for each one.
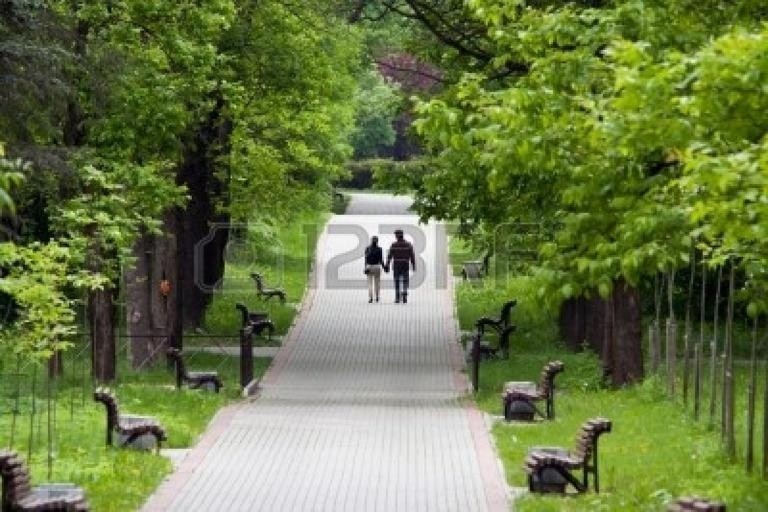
[364,236,385,303]
[385,230,415,304]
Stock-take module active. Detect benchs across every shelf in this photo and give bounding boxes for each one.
[0,450,88,511]
[469,299,613,494]
[92,272,286,455]
